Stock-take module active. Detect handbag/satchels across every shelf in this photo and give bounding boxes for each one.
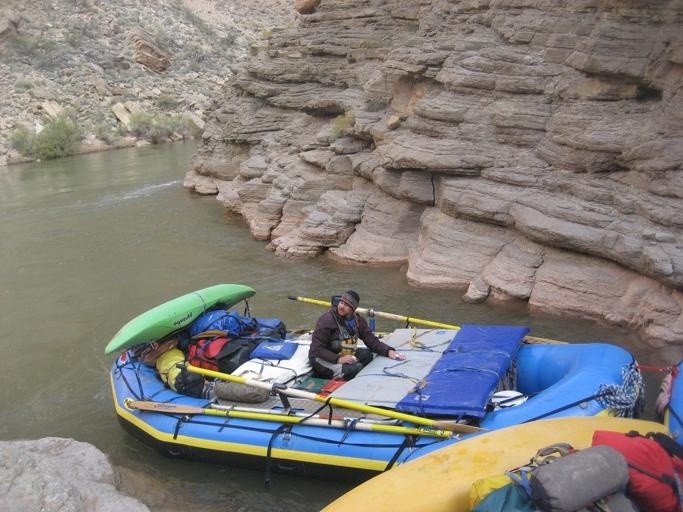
[179,310,243,349]
[238,314,287,340]
[154,349,205,397]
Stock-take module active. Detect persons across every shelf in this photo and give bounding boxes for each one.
[309,289,407,381]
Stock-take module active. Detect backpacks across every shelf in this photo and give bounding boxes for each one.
[186,338,256,375]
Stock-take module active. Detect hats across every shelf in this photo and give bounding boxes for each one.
[337,290,359,312]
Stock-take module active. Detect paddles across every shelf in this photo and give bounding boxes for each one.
[126,400,453,438]
[175,364,485,433]
[287,295,569,344]
